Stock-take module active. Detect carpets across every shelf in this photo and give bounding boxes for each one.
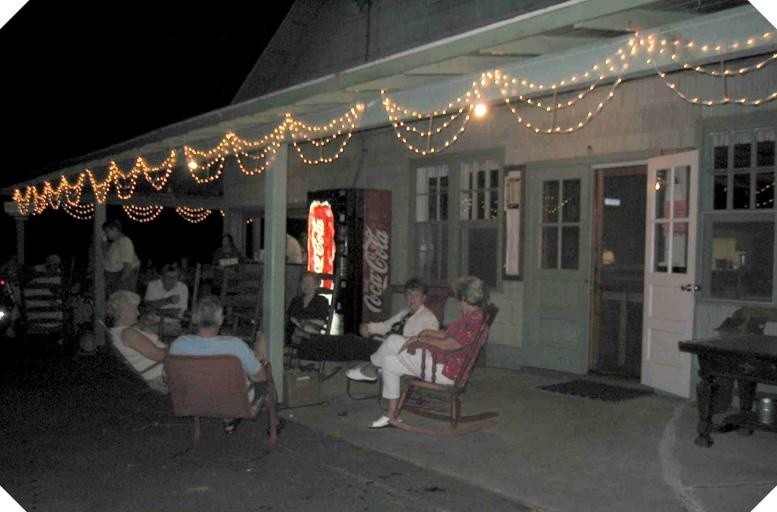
[536,379,655,405]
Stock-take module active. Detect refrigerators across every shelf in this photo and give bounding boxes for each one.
[304,189,392,335]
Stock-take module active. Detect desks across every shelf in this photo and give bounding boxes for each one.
[678,333,776,448]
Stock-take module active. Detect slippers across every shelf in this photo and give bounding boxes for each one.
[368,415,402,428]
[346,368,378,381]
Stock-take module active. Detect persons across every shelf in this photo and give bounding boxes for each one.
[346,276,486,427]
[0,219,329,438]
[359,277,439,336]
